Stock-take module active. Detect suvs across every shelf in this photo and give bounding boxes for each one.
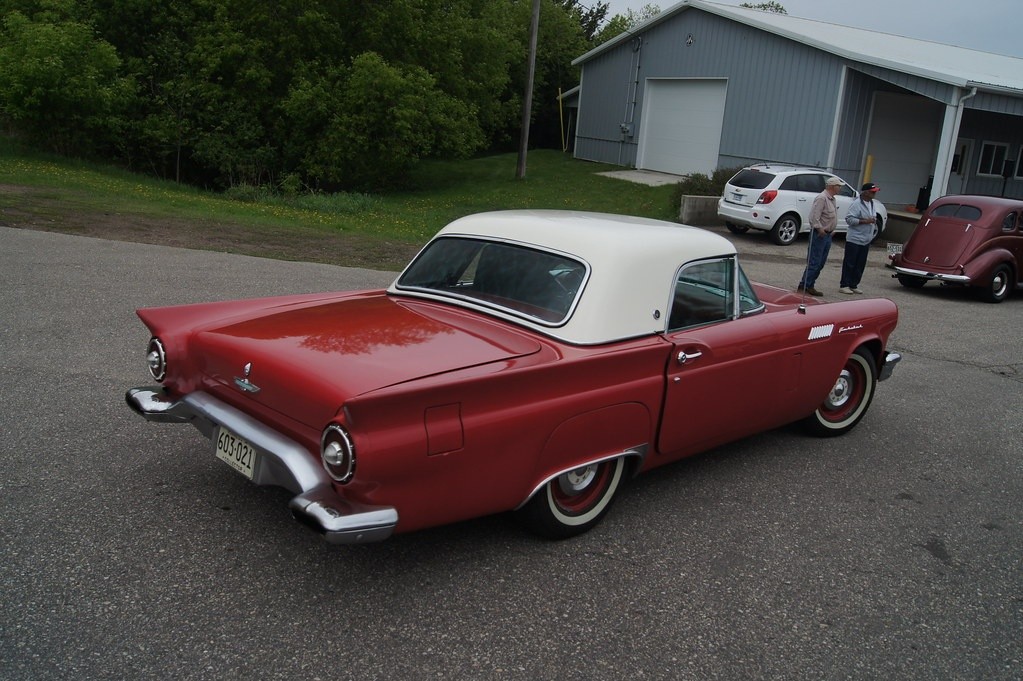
[717,162,888,247]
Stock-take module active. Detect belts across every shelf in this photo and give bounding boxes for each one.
[825,230,833,234]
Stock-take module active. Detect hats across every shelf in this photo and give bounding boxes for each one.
[861,183,880,192]
[826,177,845,186]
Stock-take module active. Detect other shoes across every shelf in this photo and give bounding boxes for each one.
[796,282,805,293]
[805,288,823,297]
[839,284,853,294]
[851,287,863,294]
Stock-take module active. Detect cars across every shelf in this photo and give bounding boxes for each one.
[125,207,904,548]
[883,194,1023,304]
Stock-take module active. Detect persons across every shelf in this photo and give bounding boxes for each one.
[837,183,883,294]
[796,177,845,297]
